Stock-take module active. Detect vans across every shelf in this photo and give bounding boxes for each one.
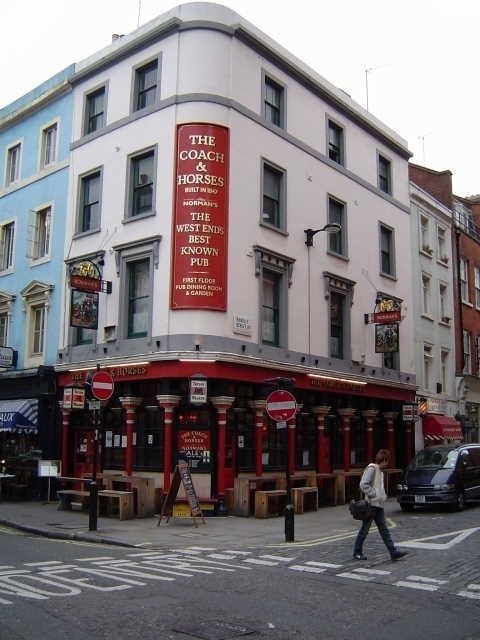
[396,442,479,511]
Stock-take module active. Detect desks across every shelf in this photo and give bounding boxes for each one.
[80,472,155,518]
[232,470,403,518]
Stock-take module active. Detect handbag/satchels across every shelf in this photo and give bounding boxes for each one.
[349,499,371,521]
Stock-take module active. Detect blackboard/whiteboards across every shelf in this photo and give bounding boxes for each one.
[179,464,202,516]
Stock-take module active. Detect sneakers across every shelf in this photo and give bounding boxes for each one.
[390,551,408,560]
[353,553,368,560]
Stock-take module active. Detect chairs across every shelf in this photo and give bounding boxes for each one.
[431,454,443,466]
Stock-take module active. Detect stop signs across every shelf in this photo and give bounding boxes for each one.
[265,390,297,422]
[91,372,114,400]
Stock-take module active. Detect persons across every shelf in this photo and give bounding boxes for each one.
[352,447,409,562]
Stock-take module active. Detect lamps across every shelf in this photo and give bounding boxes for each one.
[304,223,341,247]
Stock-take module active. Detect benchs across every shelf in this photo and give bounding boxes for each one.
[56,487,134,521]
